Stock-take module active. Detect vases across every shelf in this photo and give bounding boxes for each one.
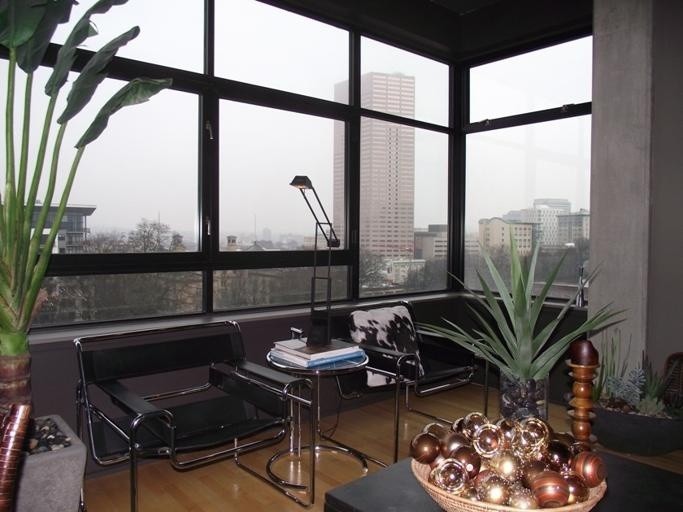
[589,404,683,456]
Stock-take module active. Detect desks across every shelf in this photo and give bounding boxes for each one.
[320,452,682,512]
[268,344,367,500]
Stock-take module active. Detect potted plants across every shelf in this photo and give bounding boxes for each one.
[411,228,623,426]
[0,0,172,512]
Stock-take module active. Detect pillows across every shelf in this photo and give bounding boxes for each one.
[346,307,425,387]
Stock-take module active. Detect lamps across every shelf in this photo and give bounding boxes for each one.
[289,175,339,346]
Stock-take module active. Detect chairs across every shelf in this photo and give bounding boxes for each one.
[68,320,311,510]
[319,295,489,470]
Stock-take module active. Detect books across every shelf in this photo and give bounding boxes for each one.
[270,338,365,368]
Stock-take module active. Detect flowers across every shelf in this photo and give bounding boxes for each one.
[580,335,680,419]
